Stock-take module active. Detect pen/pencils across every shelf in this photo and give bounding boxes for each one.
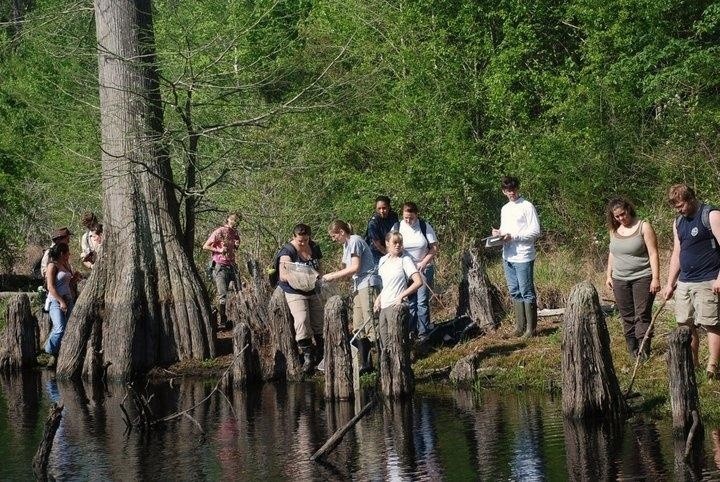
[490,225,494,229]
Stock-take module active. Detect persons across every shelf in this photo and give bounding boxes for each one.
[80,212,100,259]
[42,367,76,481]
[40,243,74,368]
[660,183,720,378]
[82,223,103,272]
[202,210,242,330]
[40,227,83,305]
[364,195,400,265]
[373,230,423,348]
[388,201,440,342]
[491,175,542,341]
[322,219,384,378]
[275,223,326,373]
[606,198,661,358]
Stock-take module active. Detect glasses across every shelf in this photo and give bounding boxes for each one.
[391,240,403,246]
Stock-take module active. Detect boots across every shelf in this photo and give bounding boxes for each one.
[356,337,373,377]
[517,302,537,341]
[502,301,526,338]
[312,333,324,366]
[297,338,315,375]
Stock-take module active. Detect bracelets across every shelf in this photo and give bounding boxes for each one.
[400,292,407,298]
[330,273,336,281]
[651,277,660,280]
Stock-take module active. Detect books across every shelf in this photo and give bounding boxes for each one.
[480,235,504,248]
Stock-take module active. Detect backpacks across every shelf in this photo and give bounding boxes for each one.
[267,241,296,289]
[31,248,54,282]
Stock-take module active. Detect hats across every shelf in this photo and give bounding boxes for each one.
[51,227,74,239]
